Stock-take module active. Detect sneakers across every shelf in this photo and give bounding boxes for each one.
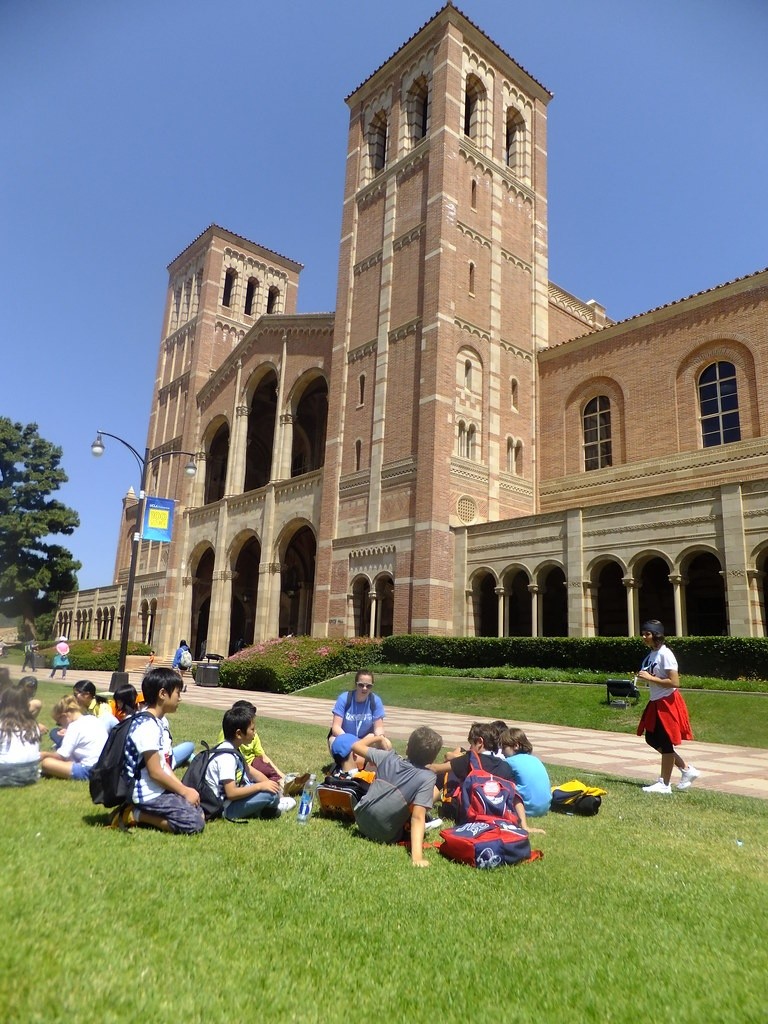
[677,765,700,790]
[642,778,672,794]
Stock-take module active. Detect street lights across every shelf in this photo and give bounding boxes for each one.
[91,431,197,693]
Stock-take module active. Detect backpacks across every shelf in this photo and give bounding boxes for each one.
[439,820,543,868]
[180,648,192,667]
[181,740,245,820]
[89,711,157,807]
[451,750,524,826]
[316,772,370,821]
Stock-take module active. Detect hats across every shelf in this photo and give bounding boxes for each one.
[59,636,67,641]
[0,636,4,641]
[331,733,361,762]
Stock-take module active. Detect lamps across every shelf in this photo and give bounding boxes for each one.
[606,680,640,705]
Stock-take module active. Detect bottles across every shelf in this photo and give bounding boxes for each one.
[296,774,317,821]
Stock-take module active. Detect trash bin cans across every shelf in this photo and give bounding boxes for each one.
[196,662,219,687]
[29,653,51,669]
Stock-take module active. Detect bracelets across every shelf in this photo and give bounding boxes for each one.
[379,734,386,738]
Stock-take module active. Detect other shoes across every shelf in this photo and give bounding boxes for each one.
[182,684,187,692]
[260,807,282,820]
[425,819,442,832]
[278,797,297,811]
[109,801,137,827]
[290,773,310,795]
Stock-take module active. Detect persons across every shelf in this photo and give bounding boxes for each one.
[22,637,39,673]
[143,651,155,673]
[203,706,297,820]
[637,620,701,793]
[171,640,192,692]
[49,636,69,679]
[283,632,294,638]
[0,668,149,787]
[327,669,392,770]
[331,721,553,835]
[110,667,205,835]
[237,639,246,652]
[200,640,207,661]
[352,726,443,867]
[217,700,286,787]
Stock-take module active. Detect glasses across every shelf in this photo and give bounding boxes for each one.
[56,712,68,726]
[75,691,89,697]
[355,682,374,690]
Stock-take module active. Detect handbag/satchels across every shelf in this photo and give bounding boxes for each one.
[550,780,607,816]
